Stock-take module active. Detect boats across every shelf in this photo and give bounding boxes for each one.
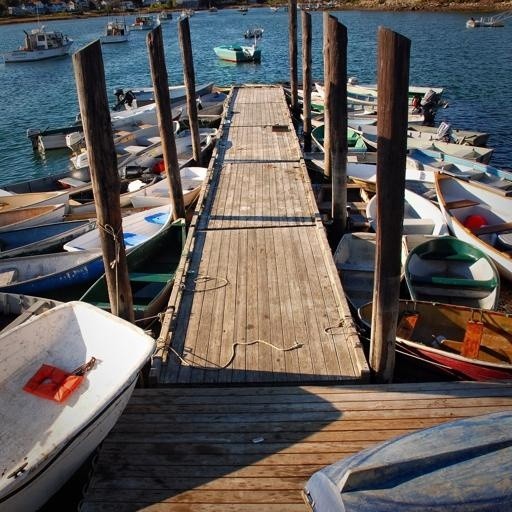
[27,82,220,149]
[126,15,157,31]
[209,7,219,12]
[171,92,227,118]
[181,104,224,127]
[0,248,106,298]
[0,152,131,201]
[70,120,179,164]
[98,13,130,43]
[298,76,441,120]
[124,128,215,166]
[208,6,218,15]
[111,83,196,108]
[237,7,248,12]
[78,218,186,327]
[242,23,264,39]
[467,10,512,27]
[283,79,445,112]
[157,12,175,23]
[99,21,131,44]
[0,292,63,338]
[115,122,181,160]
[300,111,425,127]
[360,130,494,165]
[180,9,195,18]
[300,410,512,511]
[348,118,491,148]
[1,249,105,288]
[26,103,157,153]
[244,24,264,40]
[130,14,154,31]
[159,11,173,22]
[348,168,437,198]
[111,81,215,113]
[466,16,505,28]
[349,121,494,162]
[118,127,219,180]
[65,176,157,215]
[236,9,247,13]
[178,9,196,23]
[66,105,183,173]
[315,183,371,258]
